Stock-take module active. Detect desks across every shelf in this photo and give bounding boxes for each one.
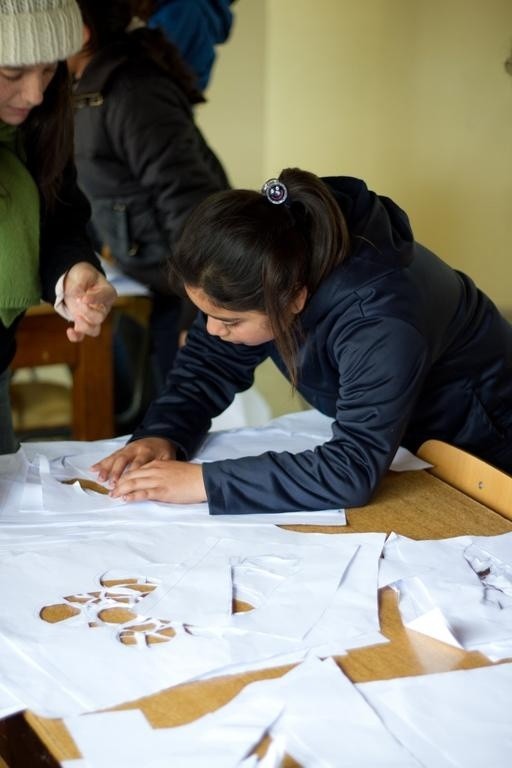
[8,252,153,441]
[21,468,512,768]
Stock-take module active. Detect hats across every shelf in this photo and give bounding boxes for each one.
[0,1,86,67]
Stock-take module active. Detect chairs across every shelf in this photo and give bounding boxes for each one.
[415,441,512,522]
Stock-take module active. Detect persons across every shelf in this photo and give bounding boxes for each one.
[1,2,120,447]
[56,0,234,432]
[92,168,511,514]
[131,0,234,90]
